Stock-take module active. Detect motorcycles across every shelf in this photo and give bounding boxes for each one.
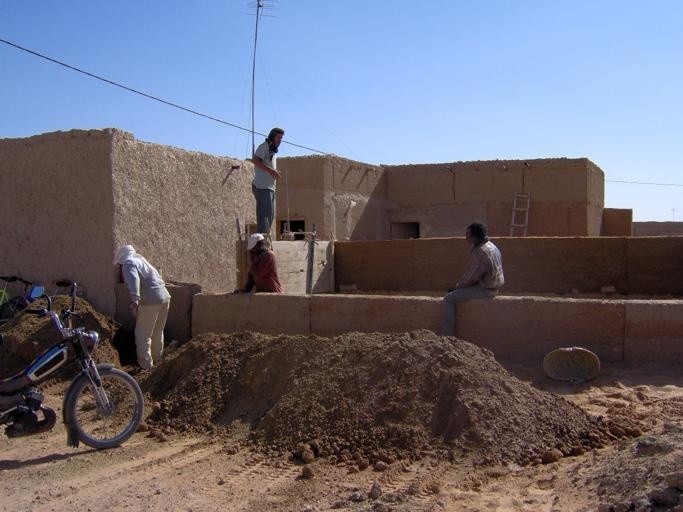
[0,278,144,449]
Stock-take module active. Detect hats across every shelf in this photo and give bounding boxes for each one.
[244,232,264,252]
[117,243,136,265]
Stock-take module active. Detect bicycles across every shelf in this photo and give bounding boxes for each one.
[0,276,73,326]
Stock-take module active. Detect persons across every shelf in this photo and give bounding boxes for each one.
[232,233,283,293]
[251,128,284,233]
[114,243,171,373]
[439,221,506,335]
